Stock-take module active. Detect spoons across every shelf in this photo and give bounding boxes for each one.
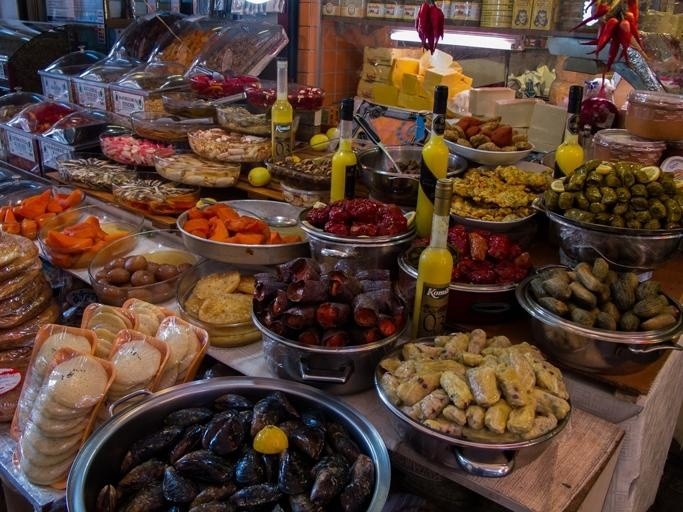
[201,198,297,229]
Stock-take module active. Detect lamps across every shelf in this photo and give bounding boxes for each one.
[385,26,528,51]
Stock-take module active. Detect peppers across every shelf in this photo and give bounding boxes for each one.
[415,2,445,56]
[569,0,645,71]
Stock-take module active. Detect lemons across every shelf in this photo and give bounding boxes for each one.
[254,425,288,455]
[249,167,270,187]
[327,127,338,140]
[195,197,217,209]
[310,134,329,151]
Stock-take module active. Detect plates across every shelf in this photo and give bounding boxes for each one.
[447,87,469,116]
[427,114,554,228]
[175,200,309,267]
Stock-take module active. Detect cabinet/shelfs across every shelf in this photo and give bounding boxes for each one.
[0,17,76,96]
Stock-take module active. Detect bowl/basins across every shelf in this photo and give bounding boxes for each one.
[358,145,470,209]
[88,228,198,303]
[176,258,267,349]
[540,184,681,268]
[3,186,89,241]
[533,197,680,270]
[98,69,325,183]
[37,205,144,271]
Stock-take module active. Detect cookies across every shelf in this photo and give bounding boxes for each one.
[12,299,209,486]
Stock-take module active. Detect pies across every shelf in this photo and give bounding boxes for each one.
[0,233,62,423]
[183,267,262,349]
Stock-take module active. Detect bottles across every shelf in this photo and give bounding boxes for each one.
[412,79,453,235]
[413,177,456,339]
[329,96,359,208]
[553,79,585,182]
[271,57,296,162]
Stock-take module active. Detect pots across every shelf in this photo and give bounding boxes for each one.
[296,206,414,268]
[516,268,682,377]
[248,282,411,395]
[373,339,573,474]
[65,375,392,512]
[397,243,536,297]
